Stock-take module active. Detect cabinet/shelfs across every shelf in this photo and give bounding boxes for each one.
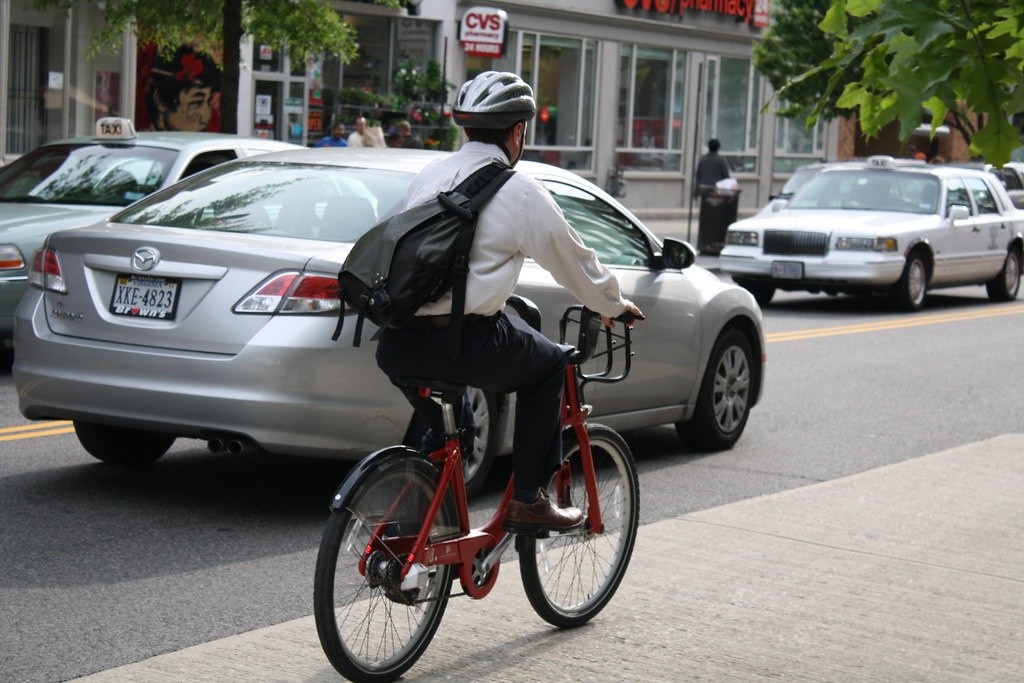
[336,102,409,137]
[407,101,453,149]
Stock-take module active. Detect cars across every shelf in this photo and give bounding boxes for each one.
[0,117,383,342]
[759,162,929,217]
[716,155,1024,311]
[12,148,769,506]
[925,163,1024,209]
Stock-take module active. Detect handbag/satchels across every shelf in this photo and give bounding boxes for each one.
[337,162,517,331]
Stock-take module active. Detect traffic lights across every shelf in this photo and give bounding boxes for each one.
[539,106,556,145]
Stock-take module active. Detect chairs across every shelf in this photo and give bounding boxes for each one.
[320,197,375,242]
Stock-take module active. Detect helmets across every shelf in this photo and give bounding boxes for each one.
[452,70,536,128]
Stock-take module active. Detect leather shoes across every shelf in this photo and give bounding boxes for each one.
[502,487,583,533]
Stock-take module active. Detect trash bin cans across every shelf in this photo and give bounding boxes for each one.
[697,184,743,257]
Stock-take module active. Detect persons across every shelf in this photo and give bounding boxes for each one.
[348,117,423,148]
[695,139,730,197]
[314,123,349,147]
[375,69,645,533]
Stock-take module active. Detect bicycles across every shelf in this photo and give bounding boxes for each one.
[313,304,647,683]
